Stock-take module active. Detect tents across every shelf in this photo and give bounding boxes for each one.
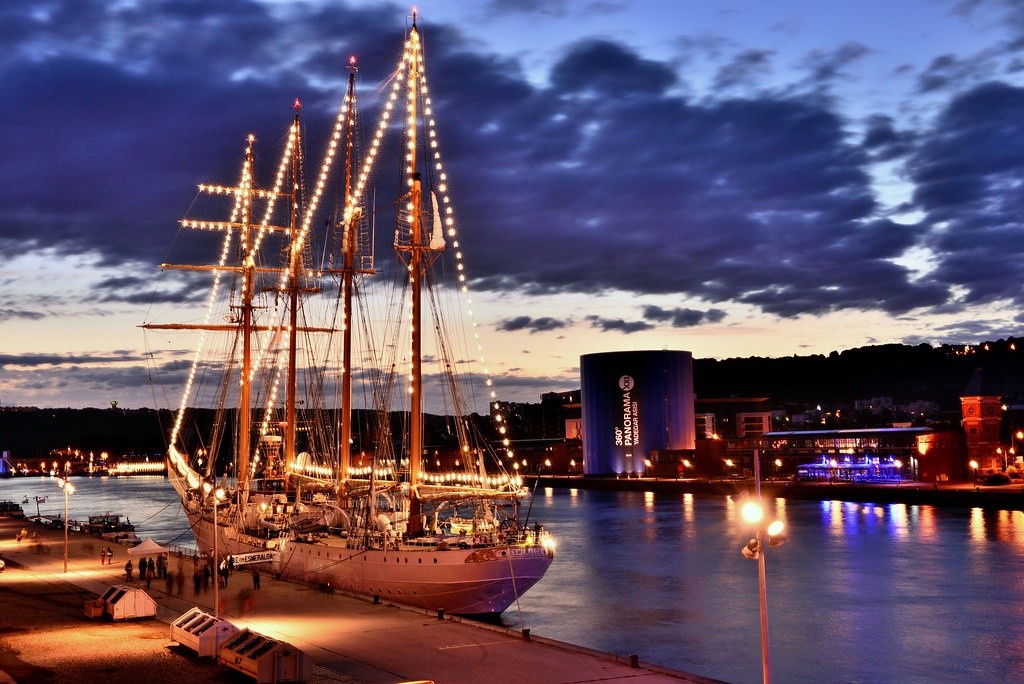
[127,537,169,572]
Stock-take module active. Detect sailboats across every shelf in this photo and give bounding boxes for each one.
[162,5,559,619]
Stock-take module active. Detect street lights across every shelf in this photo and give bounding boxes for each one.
[645,459,658,480]
[726,460,734,479]
[57,479,75,573]
[998,448,1015,471]
[775,459,781,478]
[545,460,556,477]
[1011,431,1022,463]
[970,460,979,483]
[566,460,575,478]
[683,460,689,478]
[740,501,789,684]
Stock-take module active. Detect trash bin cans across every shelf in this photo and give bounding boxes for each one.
[83,599,104,618]
[169,606,240,659]
[97,584,158,622]
[218,628,304,684]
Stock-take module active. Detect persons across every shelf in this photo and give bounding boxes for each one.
[192,547,234,596]
[251,556,261,589]
[58,513,61,518]
[125,552,168,590]
[75,520,77,529]
[101,547,113,565]
[534,521,543,542]
[16,528,27,545]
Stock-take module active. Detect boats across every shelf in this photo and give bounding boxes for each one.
[35,497,46,503]
[22,495,27,504]
[83,512,140,545]
